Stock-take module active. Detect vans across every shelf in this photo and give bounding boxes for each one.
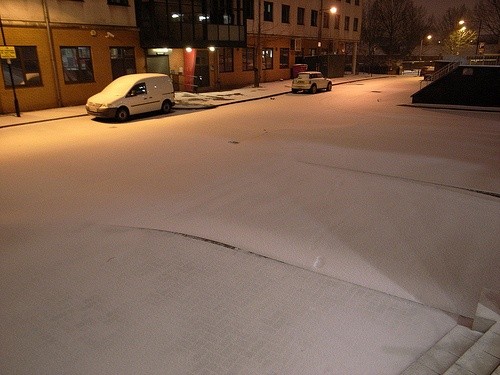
[86,73,175,121]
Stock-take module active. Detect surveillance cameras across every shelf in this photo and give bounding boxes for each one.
[106,31,114,38]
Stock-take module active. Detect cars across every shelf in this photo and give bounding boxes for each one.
[292,72,332,93]
